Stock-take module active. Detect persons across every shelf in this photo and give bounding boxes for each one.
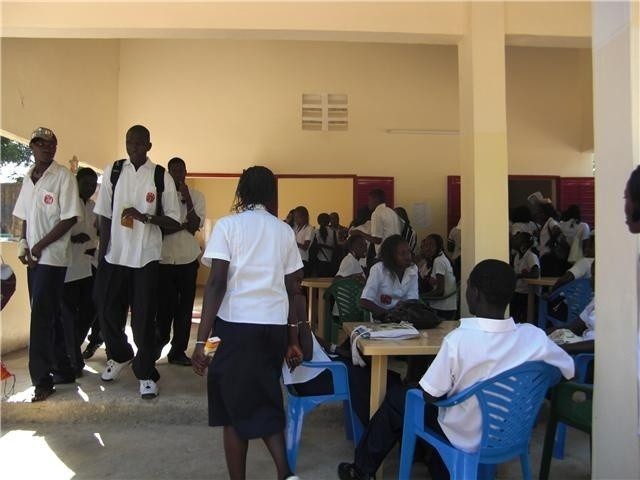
[326,238,575,480]
[621,164,640,235]
[12,127,85,403]
[93,124,182,398]
[279,172,596,403]
[152,157,206,366]
[81,317,104,361]
[60,167,102,378]
[191,165,305,480]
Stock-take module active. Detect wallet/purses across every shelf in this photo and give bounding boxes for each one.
[203,336,222,364]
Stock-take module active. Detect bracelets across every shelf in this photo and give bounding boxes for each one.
[143,214,153,226]
[29,248,41,262]
[186,205,195,217]
[195,340,206,345]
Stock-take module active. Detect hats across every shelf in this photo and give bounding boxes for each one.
[30,127,57,144]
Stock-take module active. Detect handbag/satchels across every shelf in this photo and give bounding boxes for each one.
[384,299,441,329]
[1,262,17,311]
[349,322,420,366]
[554,237,570,260]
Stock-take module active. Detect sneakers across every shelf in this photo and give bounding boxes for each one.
[101,356,135,381]
[83,340,103,359]
[139,378,159,399]
[167,347,192,366]
[338,462,376,480]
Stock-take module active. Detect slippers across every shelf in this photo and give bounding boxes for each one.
[52,377,75,384]
[32,386,56,403]
